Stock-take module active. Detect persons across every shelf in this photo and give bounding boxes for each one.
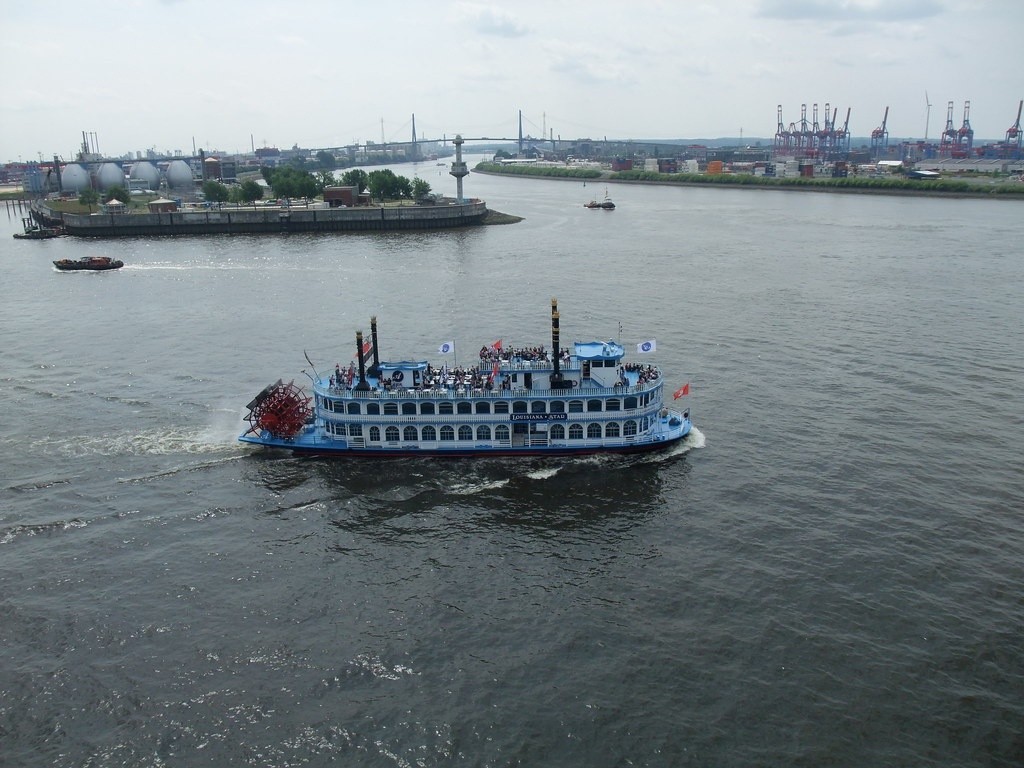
[480,345,570,366]
[619,362,659,388]
[328,361,360,391]
[383,378,391,391]
[416,363,510,390]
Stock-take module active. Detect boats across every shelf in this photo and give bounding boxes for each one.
[53,257,125,270]
[601,187,615,209]
[582,200,601,207]
[235,301,689,456]
[13,230,58,239]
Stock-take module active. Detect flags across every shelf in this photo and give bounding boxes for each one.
[438,341,454,355]
[491,362,498,379]
[637,340,656,353]
[673,383,689,400]
[355,342,371,358]
[493,340,501,349]
